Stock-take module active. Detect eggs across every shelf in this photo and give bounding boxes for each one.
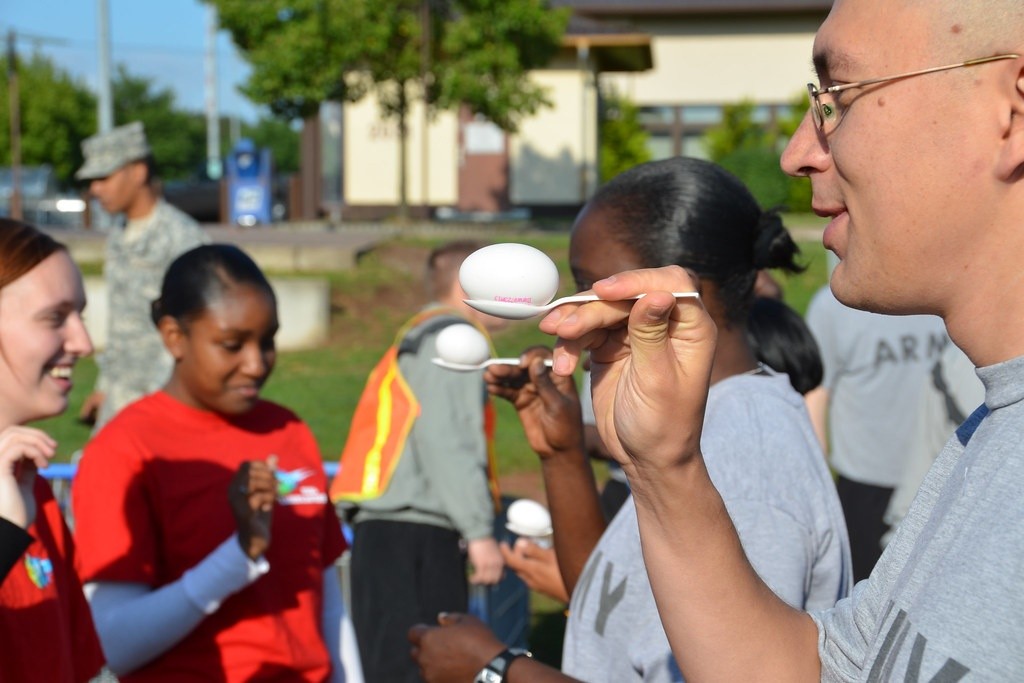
[436,322,491,366]
[507,498,551,534]
[458,242,559,307]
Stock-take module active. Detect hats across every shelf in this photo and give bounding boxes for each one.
[74,120,151,180]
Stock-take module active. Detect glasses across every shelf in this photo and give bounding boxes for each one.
[807,55,1020,130]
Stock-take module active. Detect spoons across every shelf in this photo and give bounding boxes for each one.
[429,357,554,371]
[462,293,698,319]
[505,524,555,538]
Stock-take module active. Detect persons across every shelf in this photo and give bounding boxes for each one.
[540,0,1024,683]
[0,217,115,683]
[58,243,348,683]
[328,239,528,683]
[410,156,854,683]
[73,119,214,436]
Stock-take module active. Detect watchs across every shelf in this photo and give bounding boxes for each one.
[474,647,531,683]
